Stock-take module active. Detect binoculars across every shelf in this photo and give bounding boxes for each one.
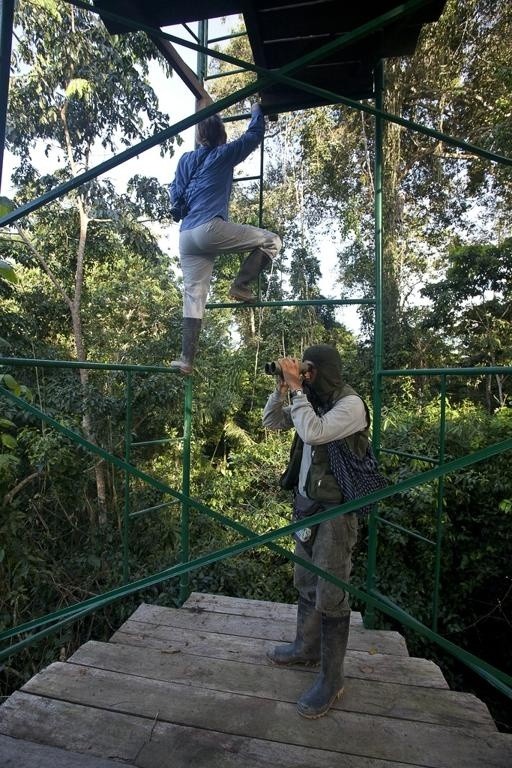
[265,361,309,375]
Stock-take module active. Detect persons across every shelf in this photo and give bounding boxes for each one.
[261,341,371,720]
[166,99,284,376]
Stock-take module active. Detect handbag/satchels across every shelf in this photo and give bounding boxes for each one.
[305,446,346,509]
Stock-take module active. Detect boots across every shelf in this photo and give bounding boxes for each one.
[229,247,273,303]
[170,316,202,375]
[296,611,350,721]
[265,596,321,665]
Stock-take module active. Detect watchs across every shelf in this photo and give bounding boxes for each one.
[289,388,304,397]
